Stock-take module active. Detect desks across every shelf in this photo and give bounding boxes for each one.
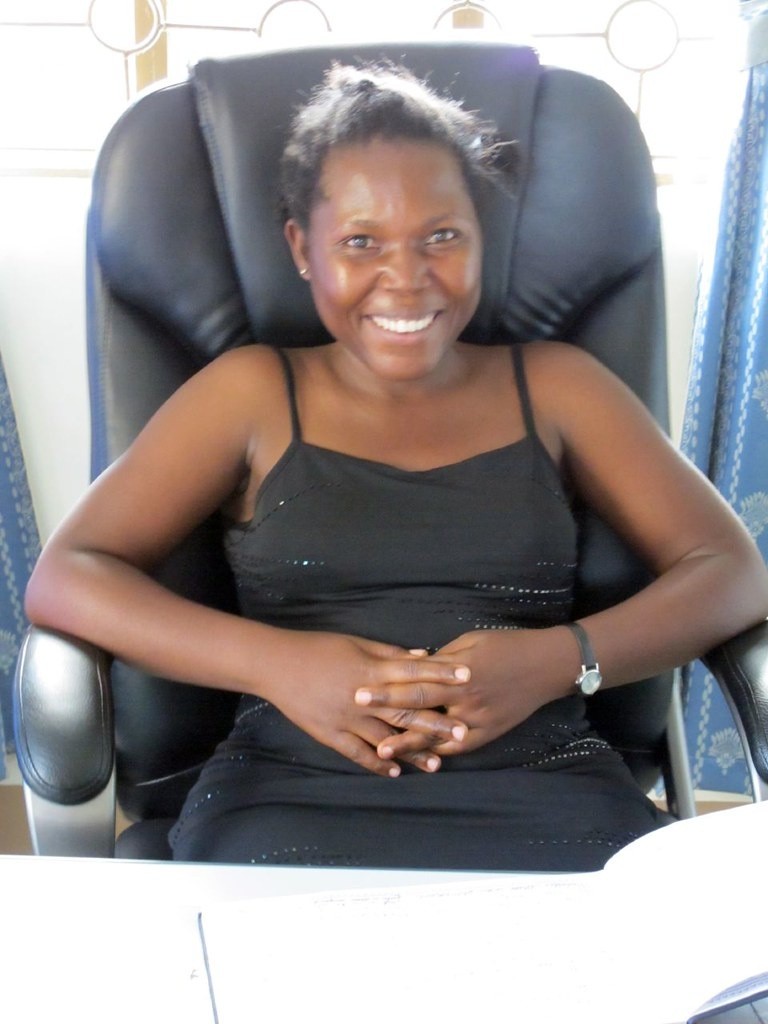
[0,850,768,1024]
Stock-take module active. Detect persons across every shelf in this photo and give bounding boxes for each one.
[27,57,767,875]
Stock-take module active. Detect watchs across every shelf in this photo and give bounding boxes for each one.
[565,621,603,700]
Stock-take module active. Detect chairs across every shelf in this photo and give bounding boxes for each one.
[9,44,765,874]
